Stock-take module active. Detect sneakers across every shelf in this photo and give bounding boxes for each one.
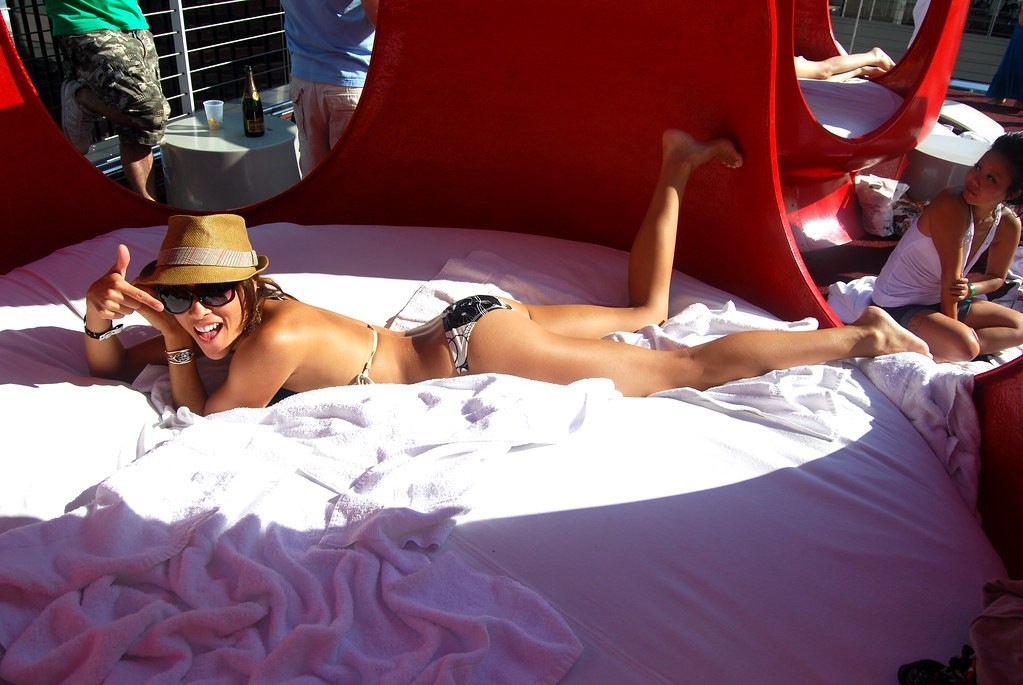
[61,78,99,156]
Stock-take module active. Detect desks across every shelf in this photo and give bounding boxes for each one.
[897,135,991,207]
[157,109,300,213]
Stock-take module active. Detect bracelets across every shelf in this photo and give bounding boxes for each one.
[84,314,123,341]
[165,347,195,365]
[963,282,976,303]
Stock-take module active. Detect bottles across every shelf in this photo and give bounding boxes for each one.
[242,65,265,137]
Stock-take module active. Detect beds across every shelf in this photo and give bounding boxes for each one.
[0,213,1012,685]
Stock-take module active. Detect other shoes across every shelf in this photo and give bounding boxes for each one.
[985,97,1006,104]
[1014,102,1023,110]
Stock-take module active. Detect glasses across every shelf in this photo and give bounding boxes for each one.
[155,280,243,314]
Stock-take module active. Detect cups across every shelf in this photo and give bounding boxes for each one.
[203,99,224,129]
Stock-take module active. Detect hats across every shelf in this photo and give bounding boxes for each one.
[139,213,269,285]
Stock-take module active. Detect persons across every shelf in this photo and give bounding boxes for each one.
[83,128,933,417]
[280,0,379,177]
[44,0,171,202]
[870,131,1023,363]
[794,48,895,82]
